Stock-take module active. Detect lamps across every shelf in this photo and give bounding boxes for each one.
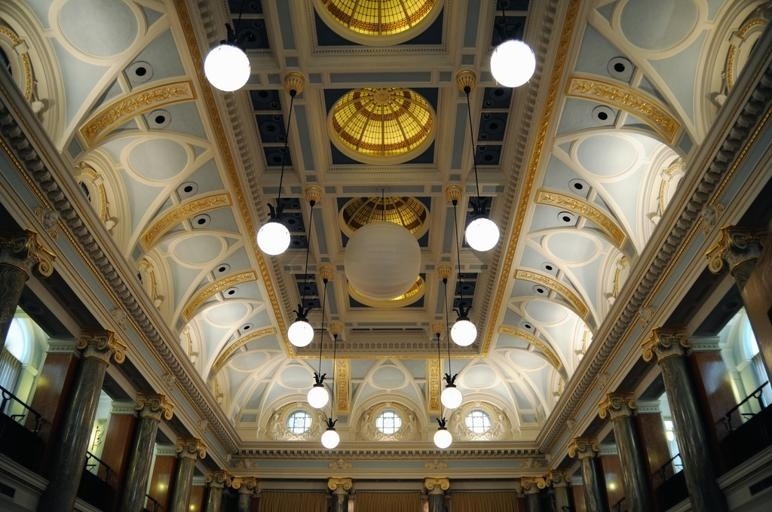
[441,277,462,409]
[344,196,421,300]
[434,332,454,449]
[287,200,317,349]
[449,199,478,346]
[490,11,535,87]
[463,85,500,252]
[320,333,340,451]
[256,89,298,256]
[203,11,251,93]
[306,277,329,409]
